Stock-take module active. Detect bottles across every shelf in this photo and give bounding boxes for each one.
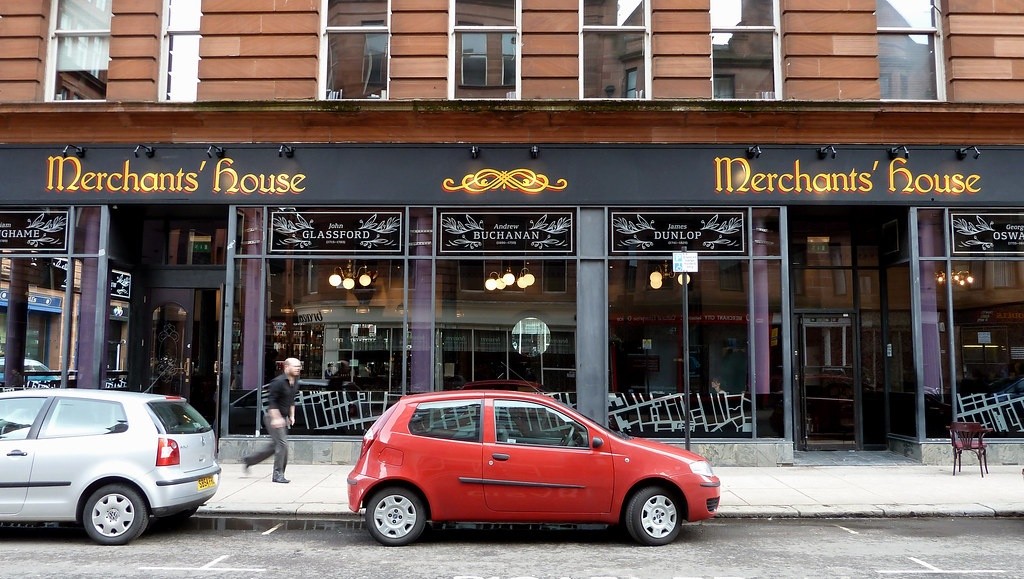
[272,322,324,379]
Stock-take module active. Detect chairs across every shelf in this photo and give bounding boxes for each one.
[945,422,994,477]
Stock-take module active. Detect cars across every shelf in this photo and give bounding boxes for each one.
[346,390,720,547]
[988,378,1024,409]
[769,375,877,439]
[213,377,364,436]
[458,380,553,391]
[0,356,58,382]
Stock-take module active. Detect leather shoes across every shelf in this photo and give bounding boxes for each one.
[243,457,249,473]
[273,477,291,483]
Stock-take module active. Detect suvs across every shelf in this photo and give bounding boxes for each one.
[0,389,222,545]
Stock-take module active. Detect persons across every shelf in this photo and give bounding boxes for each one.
[710,376,722,395]
[325,361,335,379]
[244,358,302,482]
[326,360,349,390]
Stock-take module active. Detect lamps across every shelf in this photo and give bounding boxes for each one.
[134,144,154,157]
[958,145,981,160]
[470,145,479,159]
[207,144,224,160]
[889,145,910,159]
[747,145,762,158]
[935,267,975,286]
[485,220,537,290]
[817,145,838,160]
[329,218,379,290]
[278,145,295,158]
[531,145,539,157]
[62,145,85,158]
[650,261,691,288]
